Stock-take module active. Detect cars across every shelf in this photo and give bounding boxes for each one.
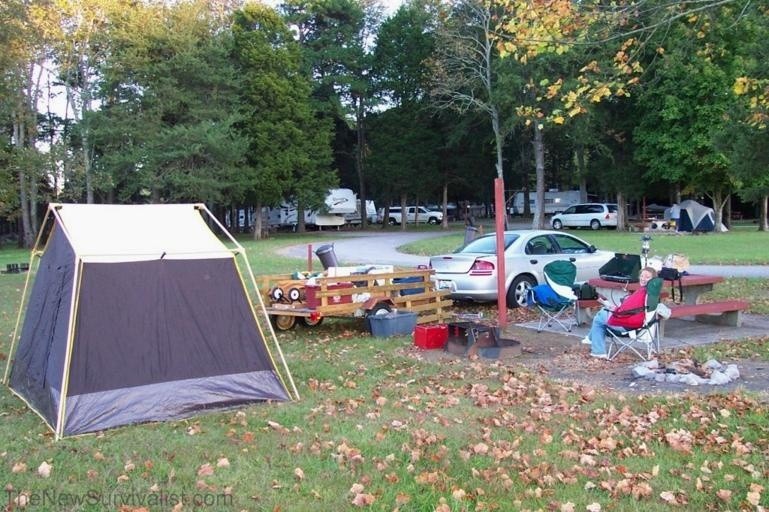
[429,230,617,308]
[550,203,619,231]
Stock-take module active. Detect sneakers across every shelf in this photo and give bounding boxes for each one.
[590,353,607,358]
[582,336,592,344]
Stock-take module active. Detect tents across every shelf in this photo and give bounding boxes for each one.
[3,204,300,444]
[664,199,729,232]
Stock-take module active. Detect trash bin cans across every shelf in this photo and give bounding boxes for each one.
[312,243,339,271]
[464,225,478,245]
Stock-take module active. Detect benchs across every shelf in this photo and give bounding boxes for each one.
[575,291,748,340]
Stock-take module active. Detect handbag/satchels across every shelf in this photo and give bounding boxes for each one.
[658,267,678,280]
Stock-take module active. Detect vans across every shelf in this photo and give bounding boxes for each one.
[381,206,443,225]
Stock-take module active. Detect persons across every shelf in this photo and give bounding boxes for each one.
[670,201,681,232]
[581,267,656,358]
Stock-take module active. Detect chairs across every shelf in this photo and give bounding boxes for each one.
[532,242,548,254]
[525,259,584,332]
[602,277,665,362]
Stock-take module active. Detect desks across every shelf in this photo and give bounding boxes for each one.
[587,273,724,337]
